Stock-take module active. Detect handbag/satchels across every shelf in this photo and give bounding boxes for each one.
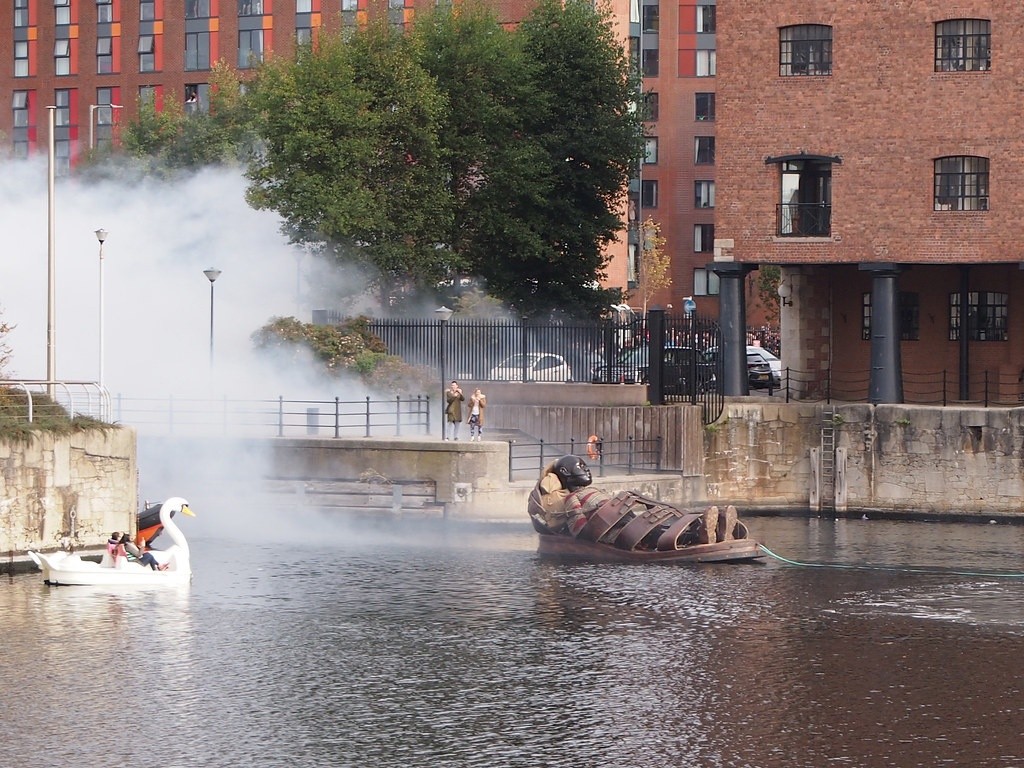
[444,405,449,414]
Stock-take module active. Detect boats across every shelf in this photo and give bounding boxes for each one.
[138,494,171,551]
[28,491,197,594]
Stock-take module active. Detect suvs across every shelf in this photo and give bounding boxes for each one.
[699,345,771,392]
[587,343,717,397]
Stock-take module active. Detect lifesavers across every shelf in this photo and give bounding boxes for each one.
[587,435,601,460]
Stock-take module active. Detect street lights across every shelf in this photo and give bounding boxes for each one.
[93,227,110,395]
[434,306,452,441]
[202,266,224,376]
[85,103,121,165]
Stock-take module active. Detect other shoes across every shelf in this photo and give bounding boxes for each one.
[477,436,481,442]
[445,438,448,442]
[454,438,460,441]
[470,436,474,441]
[157,561,170,571]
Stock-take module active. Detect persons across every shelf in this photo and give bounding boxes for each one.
[634,321,781,358]
[118,534,169,571]
[555,454,737,551]
[467,387,487,440]
[445,381,465,440]
[107,532,120,556]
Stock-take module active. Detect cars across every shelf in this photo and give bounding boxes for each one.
[709,344,785,384]
[488,351,573,385]
